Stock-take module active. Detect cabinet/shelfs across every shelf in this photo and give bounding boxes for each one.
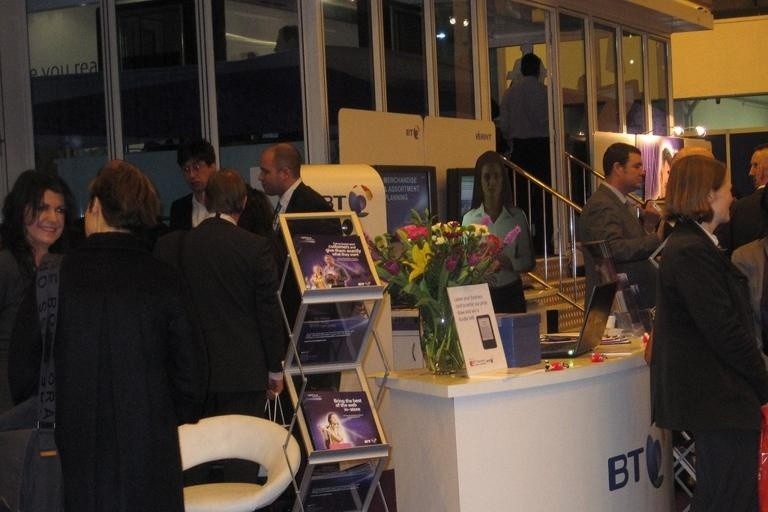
[275,254,392,511]
[391,312,528,375]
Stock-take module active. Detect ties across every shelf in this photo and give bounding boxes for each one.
[625,199,641,226]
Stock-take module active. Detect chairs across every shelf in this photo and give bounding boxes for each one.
[178,412,302,510]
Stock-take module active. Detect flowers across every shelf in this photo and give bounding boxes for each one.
[373,206,521,375]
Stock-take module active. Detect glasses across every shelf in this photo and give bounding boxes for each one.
[184,162,206,171]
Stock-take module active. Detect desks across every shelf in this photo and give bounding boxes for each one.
[373,328,679,511]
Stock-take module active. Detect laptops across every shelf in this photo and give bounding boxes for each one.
[540,280,619,359]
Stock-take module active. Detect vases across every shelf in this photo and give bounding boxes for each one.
[418,305,465,377]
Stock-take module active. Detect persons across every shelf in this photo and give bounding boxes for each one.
[495,51,550,260]
[244,24,299,60]
[576,141,666,331]
[320,409,351,448]
[176,166,288,420]
[731,176,768,370]
[0,162,77,457]
[460,148,537,312]
[169,133,218,228]
[309,262,329,288]
[320,252,351,288]
[257,141,346,395]
[238,188,284,267]
[713,141,768,251]
[646,151,768,512]
[0,156,179,512]
